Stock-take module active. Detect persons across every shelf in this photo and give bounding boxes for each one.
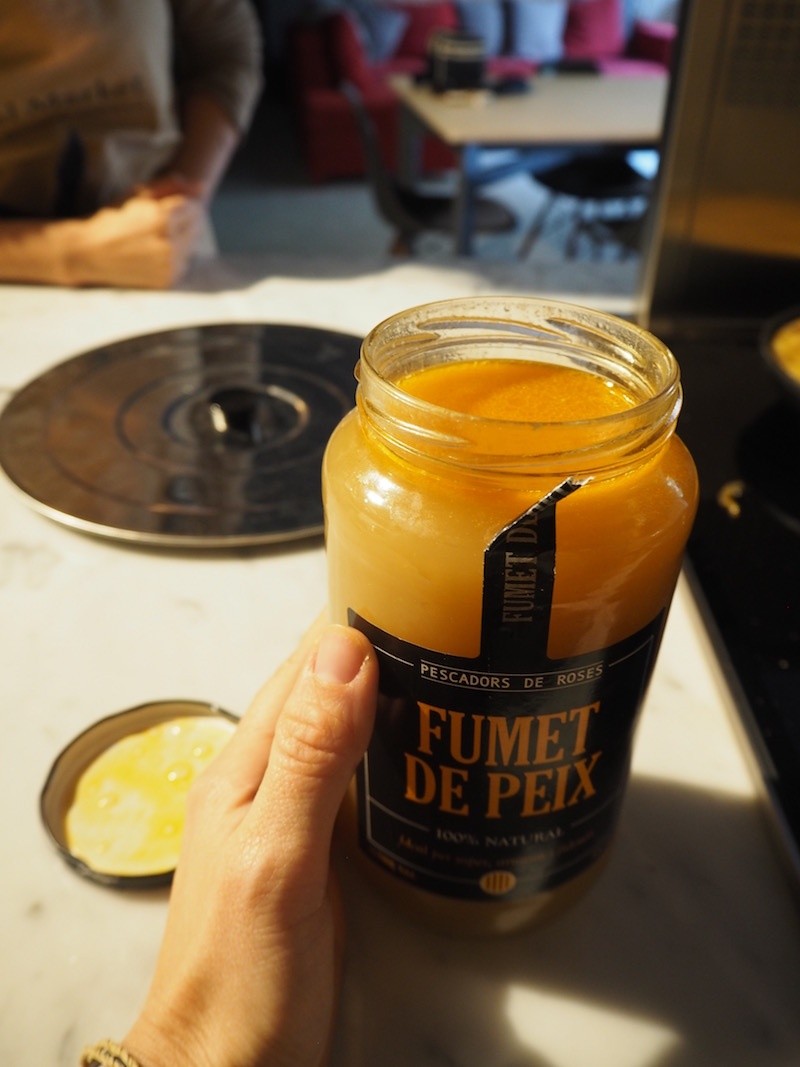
[0,0,264,252]
[82,613,380,1067]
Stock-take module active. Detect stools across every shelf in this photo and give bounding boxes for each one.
[515,156,656,267]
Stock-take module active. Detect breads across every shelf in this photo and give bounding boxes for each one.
[1,193,199,288]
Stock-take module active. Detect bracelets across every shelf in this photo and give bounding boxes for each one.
[82,1040,141,1067]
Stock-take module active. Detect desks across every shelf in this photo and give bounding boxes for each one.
[0,249,800,1067]
[385,61,670,258]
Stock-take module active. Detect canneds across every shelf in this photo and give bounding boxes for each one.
[321,297,702,937]
